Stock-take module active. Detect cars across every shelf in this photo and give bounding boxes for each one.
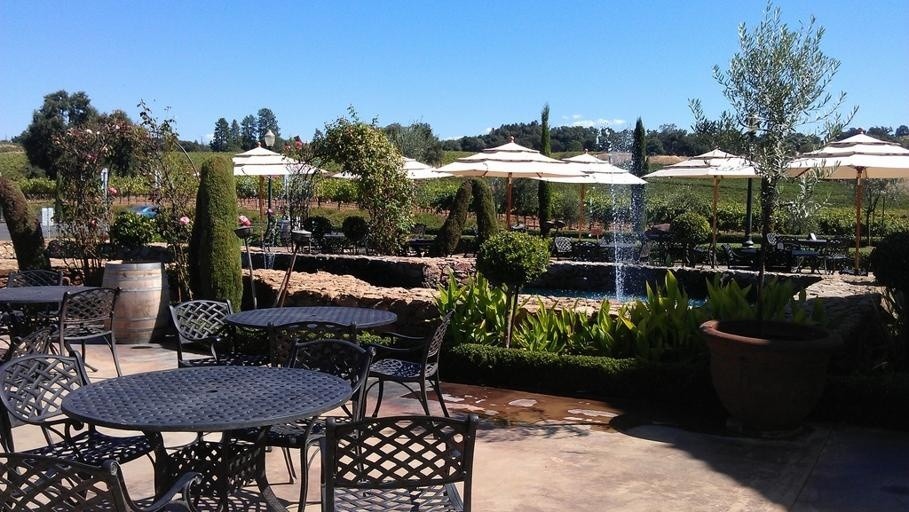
[125,203,165,221]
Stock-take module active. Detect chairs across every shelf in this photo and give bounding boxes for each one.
[224,339,371,512]
[0,349,169,511]
[319,413,479,512]
[0,269,63,360]
[0,453,205,512]
[260,321,357,369]
[360,311,454,421]
[38,287,121,385]
[168,300,236,368]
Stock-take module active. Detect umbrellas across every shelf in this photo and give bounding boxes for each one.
[639,148,765,246]
[785,130,909,274]
[193,142,328,220]
[431,136,589,230]
[529,148,648,240]
[332,153,454,181]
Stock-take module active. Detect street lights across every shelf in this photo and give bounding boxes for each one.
[263,130,275,246]
[738,111,760,250]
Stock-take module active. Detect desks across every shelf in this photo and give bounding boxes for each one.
[224,305,398,369]
[0,287,100,372]
[61,364,352,510]
